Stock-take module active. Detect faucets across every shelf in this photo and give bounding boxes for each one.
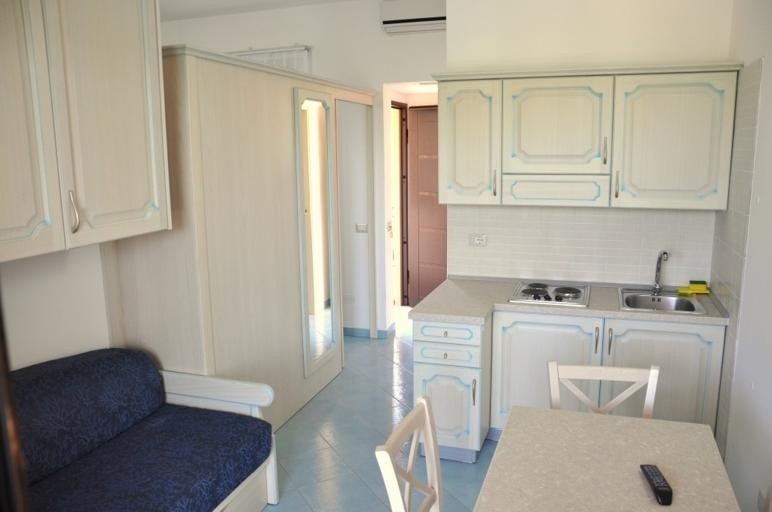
[654,250,669,285]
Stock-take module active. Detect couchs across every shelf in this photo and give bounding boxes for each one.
[9,347,280,512]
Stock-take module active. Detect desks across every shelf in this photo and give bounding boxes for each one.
[468,402,741,512]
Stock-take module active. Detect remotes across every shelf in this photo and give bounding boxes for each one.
[639,464,674,506]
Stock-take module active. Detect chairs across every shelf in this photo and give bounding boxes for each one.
[373,395,446,512]
[548,358,661,415]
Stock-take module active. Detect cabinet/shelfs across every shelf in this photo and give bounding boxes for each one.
[611,66,739,211]
[104,45,343,437]
[413,319,491,464]
[432,72,503,209]
[502,70,615,209]
[493,310,727,443]
[1,0,176,265]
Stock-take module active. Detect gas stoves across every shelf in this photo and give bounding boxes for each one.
[507,279,591,309]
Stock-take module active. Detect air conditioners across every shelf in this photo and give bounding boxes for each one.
[379,0,446,33]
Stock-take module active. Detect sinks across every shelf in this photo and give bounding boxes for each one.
[617,287,708,315]
